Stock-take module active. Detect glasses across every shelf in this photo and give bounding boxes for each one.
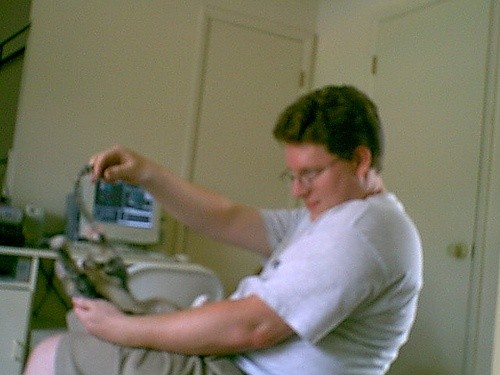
[280,155,340,186]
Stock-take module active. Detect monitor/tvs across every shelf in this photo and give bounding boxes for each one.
[75,169,163,247]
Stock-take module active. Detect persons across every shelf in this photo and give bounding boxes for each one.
[17,82,424,375]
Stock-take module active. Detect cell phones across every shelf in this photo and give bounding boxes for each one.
[58,247,106,299]
